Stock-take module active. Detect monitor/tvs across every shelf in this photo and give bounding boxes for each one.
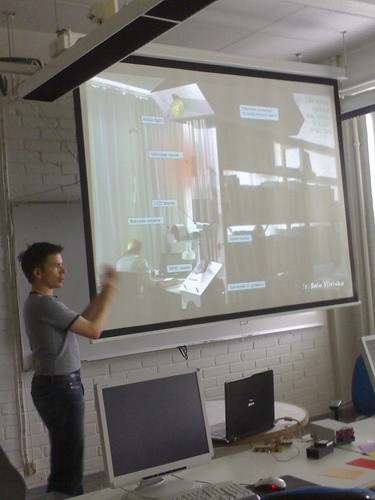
[358,334,375,396]
[92,368,213,495]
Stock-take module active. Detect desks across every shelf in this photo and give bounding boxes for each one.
[204,399,309,449]
[66,417,375,500]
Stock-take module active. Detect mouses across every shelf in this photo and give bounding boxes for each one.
[252,475,288,490]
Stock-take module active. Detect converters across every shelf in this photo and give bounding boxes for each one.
[305,442,334,460]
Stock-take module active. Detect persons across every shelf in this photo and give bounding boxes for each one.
[116,240,155,299]
[17,241,118,500]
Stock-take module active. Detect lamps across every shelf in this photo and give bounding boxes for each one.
[339,89,375,121]
[17,0,217,102]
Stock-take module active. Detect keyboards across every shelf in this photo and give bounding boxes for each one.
[147,479,261,500]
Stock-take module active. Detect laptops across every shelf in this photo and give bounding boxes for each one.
[210,369,276,444]
